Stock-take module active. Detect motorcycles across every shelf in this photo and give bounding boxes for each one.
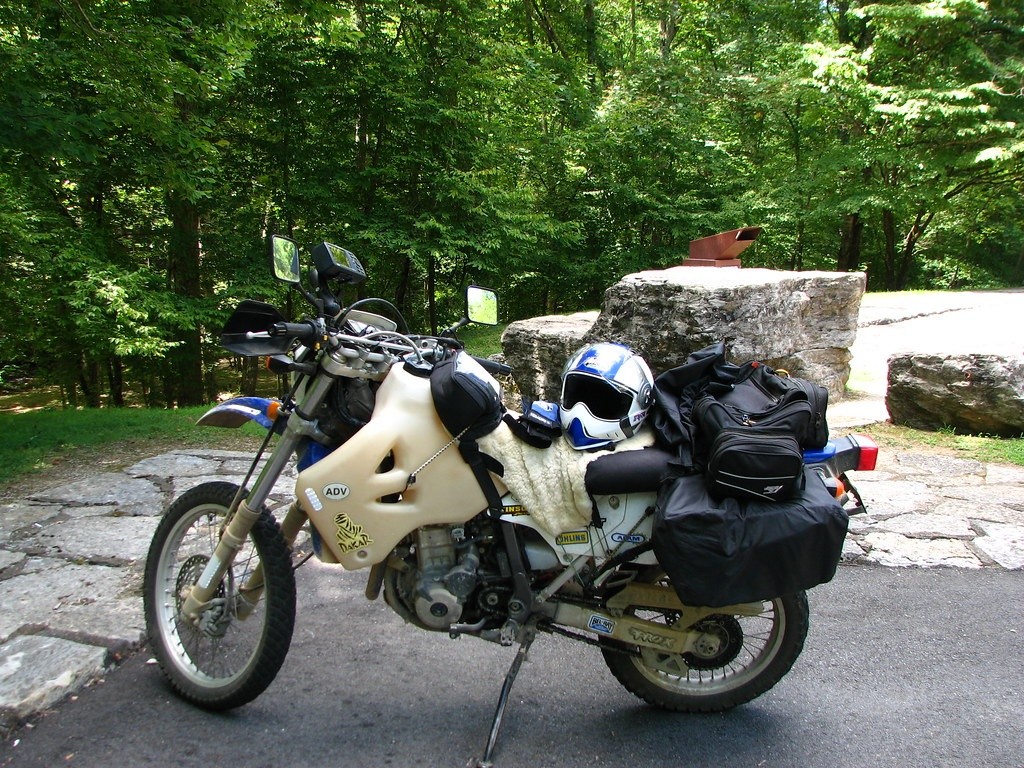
[141,234,878,768]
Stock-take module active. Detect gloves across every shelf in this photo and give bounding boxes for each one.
[521,400,558,448]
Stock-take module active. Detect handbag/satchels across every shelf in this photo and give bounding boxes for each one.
[650,464,848,608]
[690,358,829,504]
[430,348,508,443]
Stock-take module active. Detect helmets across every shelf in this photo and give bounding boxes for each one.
[559,341,655,451]
